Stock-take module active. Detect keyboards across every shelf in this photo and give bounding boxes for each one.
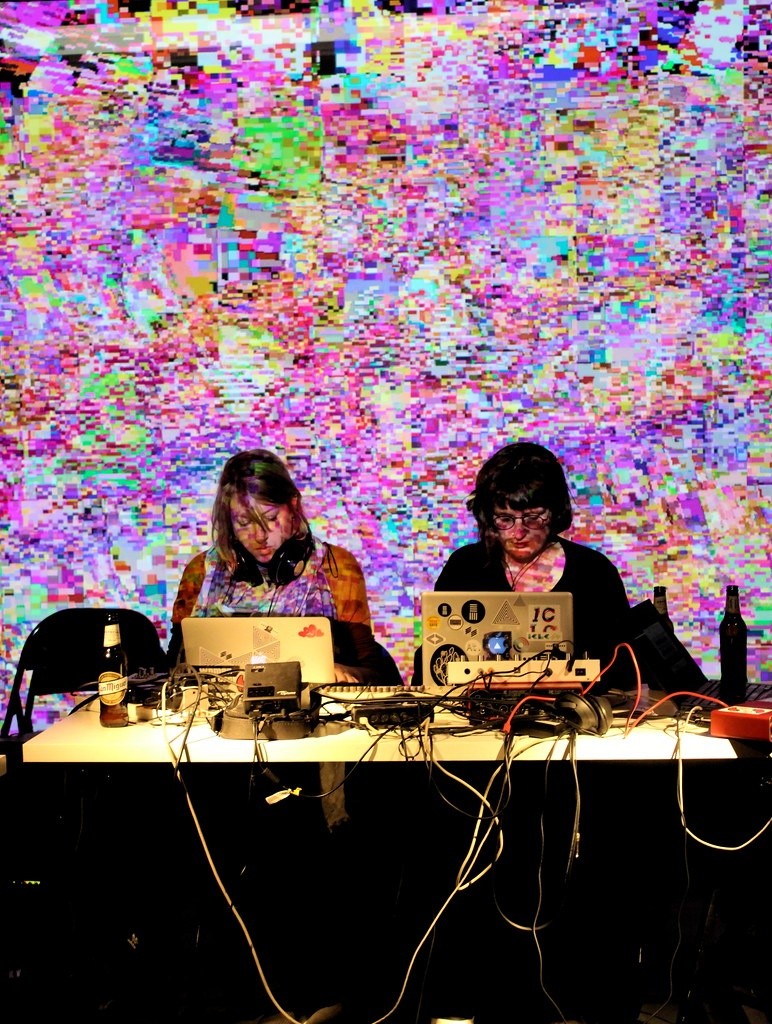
[318,685,438,700]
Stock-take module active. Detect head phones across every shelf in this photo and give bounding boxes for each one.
[226,509,314,583]
[557,691,615,735]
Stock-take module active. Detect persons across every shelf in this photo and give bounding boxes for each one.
[165,448,377,685]
[408,442,640,687]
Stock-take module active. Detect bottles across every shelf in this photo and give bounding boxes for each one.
[716,583,750,701]
[99,611,132,728]
[651,586,676,633]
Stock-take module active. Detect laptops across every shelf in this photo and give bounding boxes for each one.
[181,617,335,683]
[422,590,574,697]
[616,597,771,706]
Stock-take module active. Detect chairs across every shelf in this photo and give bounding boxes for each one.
[0,607,166,768]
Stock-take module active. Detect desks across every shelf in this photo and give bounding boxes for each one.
[20,691,769,764]
